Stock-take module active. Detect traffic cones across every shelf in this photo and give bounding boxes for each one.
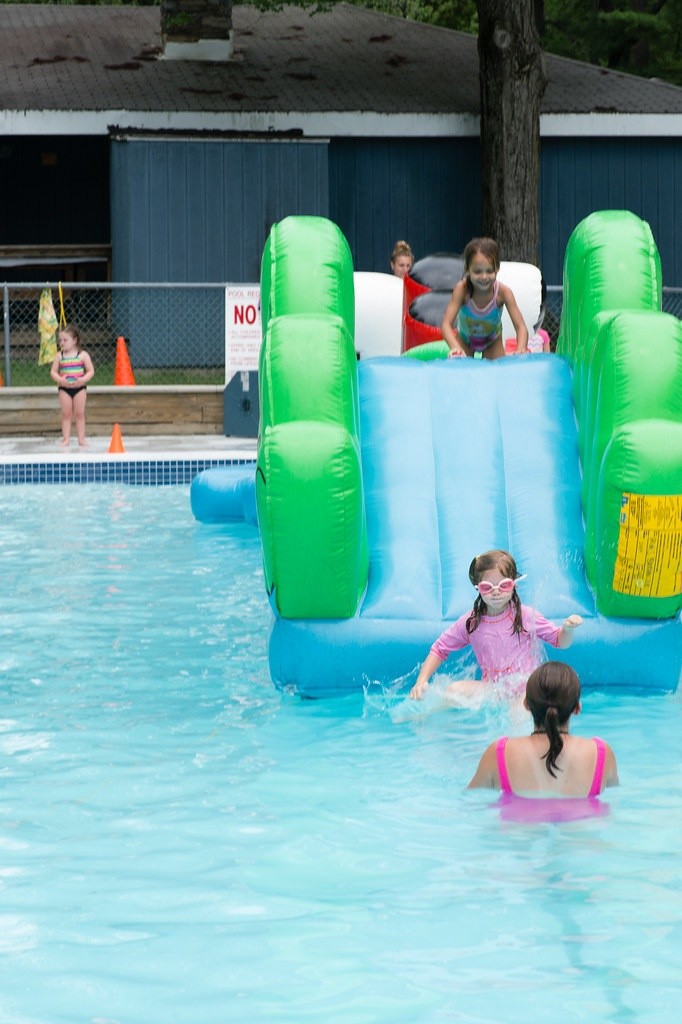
[110,336,137,385]
[108,423,124,453]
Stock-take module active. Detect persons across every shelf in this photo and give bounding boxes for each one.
[442,237,529,356]
[51,327,94,447]
[409,550,583,716]
[390,240,413,277]
[468,661,619,821]
[529,327,552,352]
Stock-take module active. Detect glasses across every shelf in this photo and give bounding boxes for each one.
[474,579,515,594]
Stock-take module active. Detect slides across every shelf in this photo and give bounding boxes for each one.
[256,209,682,702]
